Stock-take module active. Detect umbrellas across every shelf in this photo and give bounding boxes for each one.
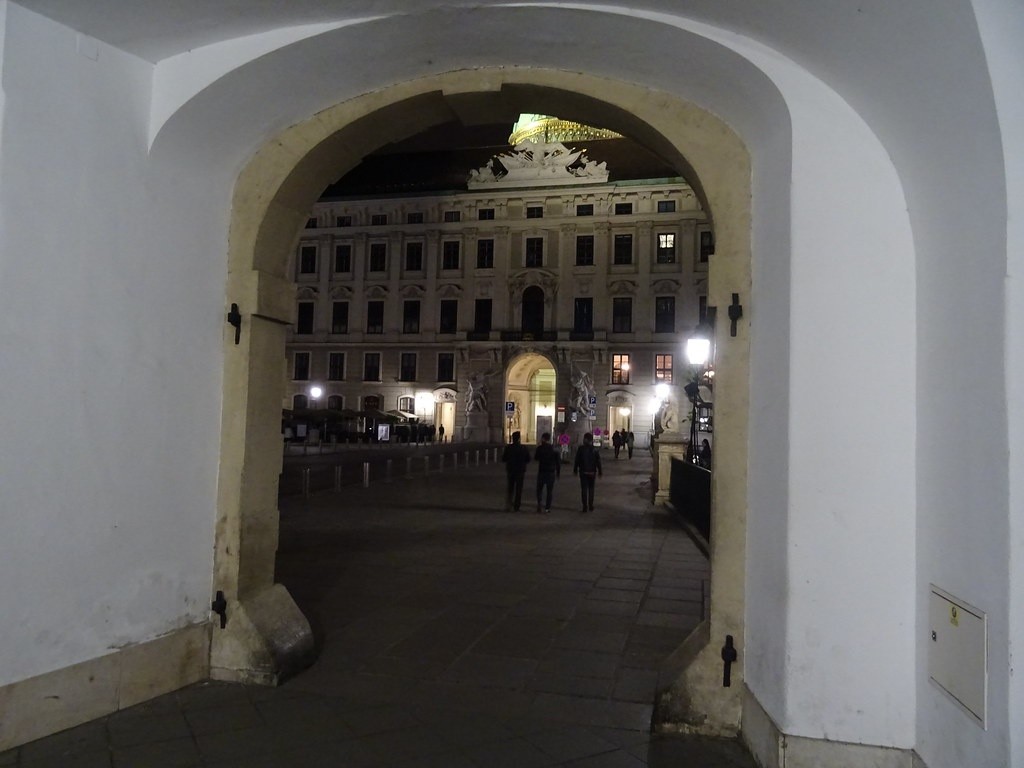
[282,408,421,441]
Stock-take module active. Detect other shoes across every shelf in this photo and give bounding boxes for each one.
[583,507,587,513]
[505,505,511,511]
[615,455,618,458]
[545,509,550,512]
[514,505,519,512]
[589,506,594,512]
[537,507,542,513]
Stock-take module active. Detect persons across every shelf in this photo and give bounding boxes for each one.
[569,368,594,417]
[396,417,444,446]
[532,432,562,515]
[501,430,531,513]
[699,439,711,471]
[465,373,490,413]
[612,427,634,460]
[573,432,601,513]
